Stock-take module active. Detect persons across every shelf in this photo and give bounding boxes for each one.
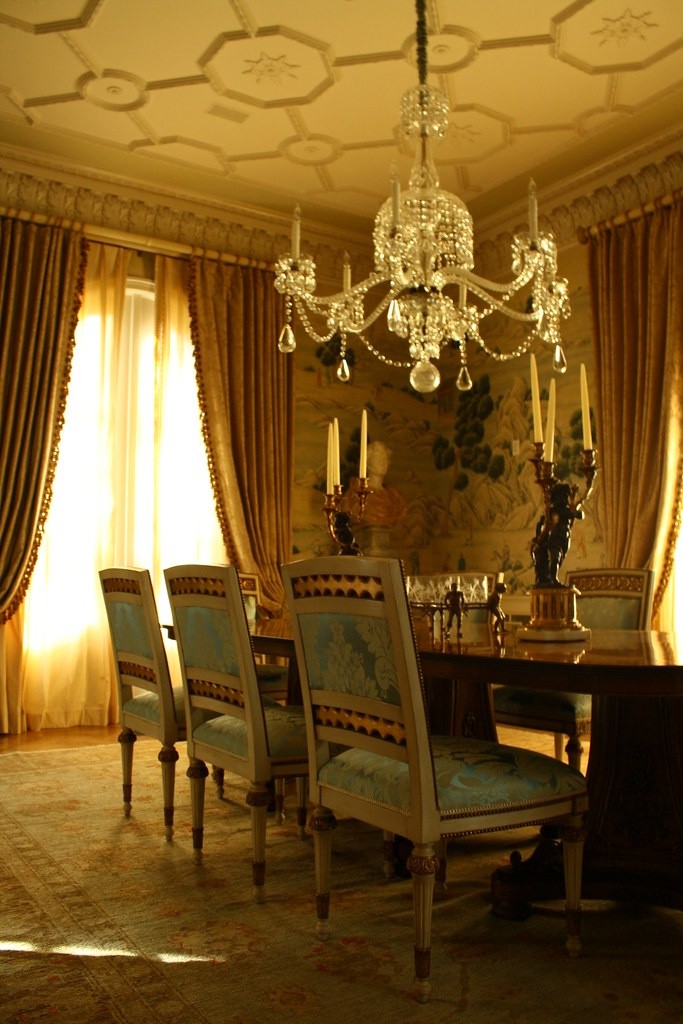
[488,583,509,633]
[445,584,464,635]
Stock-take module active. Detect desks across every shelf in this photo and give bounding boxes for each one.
[163,618,683,919]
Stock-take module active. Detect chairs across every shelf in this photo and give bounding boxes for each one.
[280,555,589,1002]
[163,562,394,904]
[403,572,490,620]
[491,568,653,769]
[237,573,288,701]
[98,567,285,841]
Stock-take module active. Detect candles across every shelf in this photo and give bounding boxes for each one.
[580,364,594,451]
[529,352,543,442]
[334,418,340,485]
[326,423,334,495]
[359,408,367,479]
[544,378,555,462]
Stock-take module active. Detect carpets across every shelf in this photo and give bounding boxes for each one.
[0,727,683,1024]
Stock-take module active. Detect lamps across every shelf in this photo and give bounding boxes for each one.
[272,0,572,395]
